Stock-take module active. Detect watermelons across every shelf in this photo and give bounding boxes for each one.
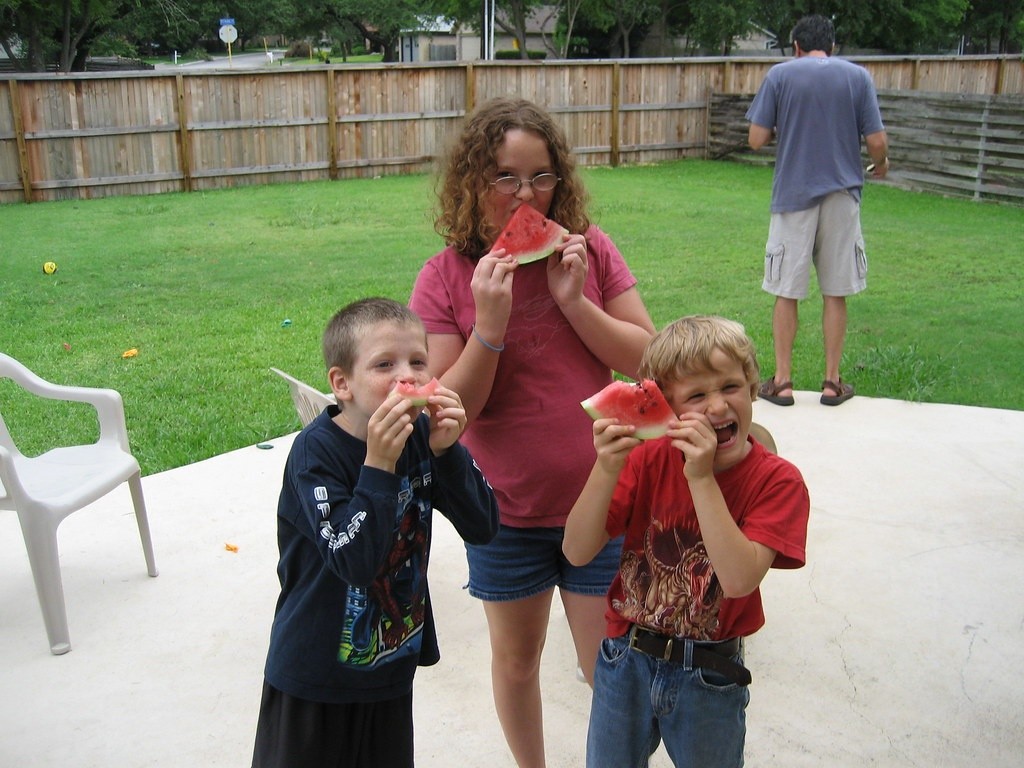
[385,377,442,408]
[580,378,679,439]
[488,203,570,264]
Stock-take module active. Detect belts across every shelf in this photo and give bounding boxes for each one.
[620,622,751,687]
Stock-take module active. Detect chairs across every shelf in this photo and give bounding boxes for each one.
[0,352,158,656]
[269,366,340,433]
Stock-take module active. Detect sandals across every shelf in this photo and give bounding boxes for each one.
[757,376,794,406]
[820,377,855,406]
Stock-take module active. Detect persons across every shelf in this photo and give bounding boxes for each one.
[562,315,809,768]
[743,13,890,406]
[250,296,500,768]
[410,96,656,768]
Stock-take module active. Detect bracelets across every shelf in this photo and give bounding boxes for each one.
[473,326,504,351]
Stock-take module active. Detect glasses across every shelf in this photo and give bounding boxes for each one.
[478,172,562,195]
